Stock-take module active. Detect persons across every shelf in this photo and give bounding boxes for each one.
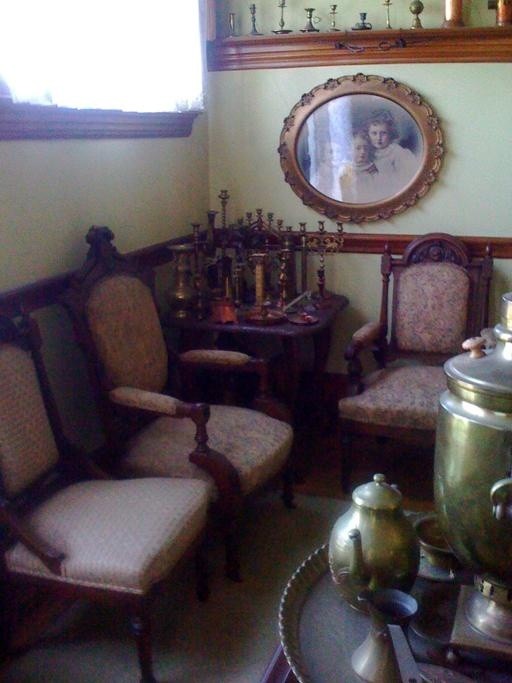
[365,106,420,204]
[336,126,376,204]
[307,136,342,202]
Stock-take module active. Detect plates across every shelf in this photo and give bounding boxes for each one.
[243,308,284,327]
[287,314,319,327]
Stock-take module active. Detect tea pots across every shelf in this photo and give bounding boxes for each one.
[326,472,421,618]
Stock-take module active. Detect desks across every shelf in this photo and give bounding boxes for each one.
[161,284,347,417]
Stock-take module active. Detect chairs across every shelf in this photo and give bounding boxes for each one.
[336,231,501,494]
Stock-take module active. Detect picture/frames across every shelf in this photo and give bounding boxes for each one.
[276,71,448,221]
